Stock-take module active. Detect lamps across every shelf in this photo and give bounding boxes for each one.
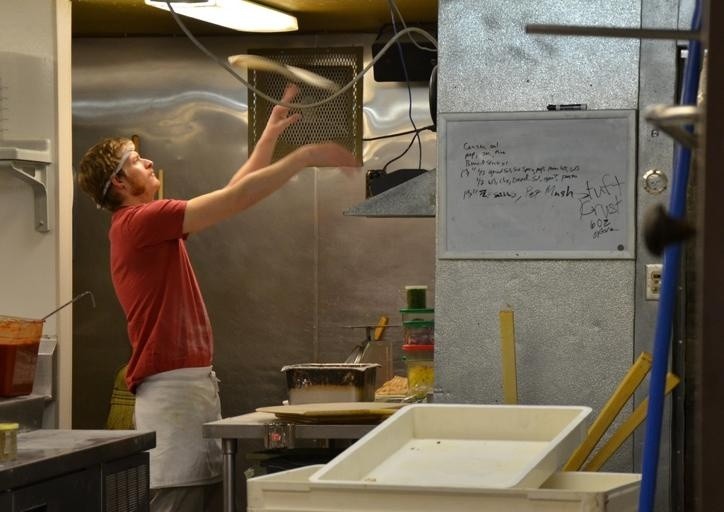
[145,0,298,34]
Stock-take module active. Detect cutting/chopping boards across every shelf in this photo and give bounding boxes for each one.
[253,399,408,417]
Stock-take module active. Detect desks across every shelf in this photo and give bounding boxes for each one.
[2,430,158,511]
[202,411,378,512]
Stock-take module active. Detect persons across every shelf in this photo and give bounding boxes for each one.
[77,81,358,511]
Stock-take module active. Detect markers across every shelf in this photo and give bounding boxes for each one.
[547,104,587,110]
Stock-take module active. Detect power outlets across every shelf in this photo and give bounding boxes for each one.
[647,263,663,300]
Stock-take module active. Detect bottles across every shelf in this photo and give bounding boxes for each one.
[404,284,427,309]
